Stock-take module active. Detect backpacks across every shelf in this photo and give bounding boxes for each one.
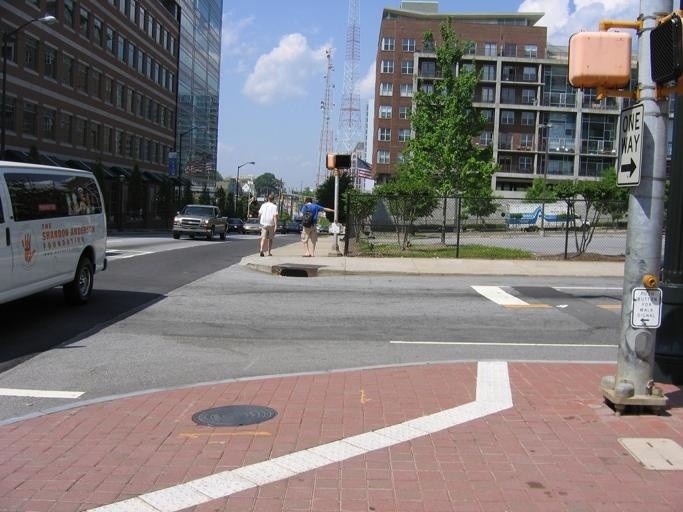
[301,205,313,228]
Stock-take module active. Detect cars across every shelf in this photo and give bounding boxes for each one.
[226,217,329,235]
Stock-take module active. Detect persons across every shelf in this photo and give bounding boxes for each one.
[257,195,278,256]
[299,198,334,256]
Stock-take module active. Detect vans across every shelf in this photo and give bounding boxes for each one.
[0,161,107,309]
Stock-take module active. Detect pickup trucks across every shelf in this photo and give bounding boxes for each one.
[173,204,229,239]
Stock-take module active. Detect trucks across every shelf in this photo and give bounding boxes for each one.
[505,201,590,231]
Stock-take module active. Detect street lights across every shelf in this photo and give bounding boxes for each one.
[178,125,199,213]
[1,15,55,160]
[535,123,553,230]
[235,162,255,216]
[326,154,351,170]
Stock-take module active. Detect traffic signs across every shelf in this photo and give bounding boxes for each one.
[631,287,663,329]
[612,104,644,188]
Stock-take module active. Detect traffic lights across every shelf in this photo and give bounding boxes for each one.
[648,10,683,97]
